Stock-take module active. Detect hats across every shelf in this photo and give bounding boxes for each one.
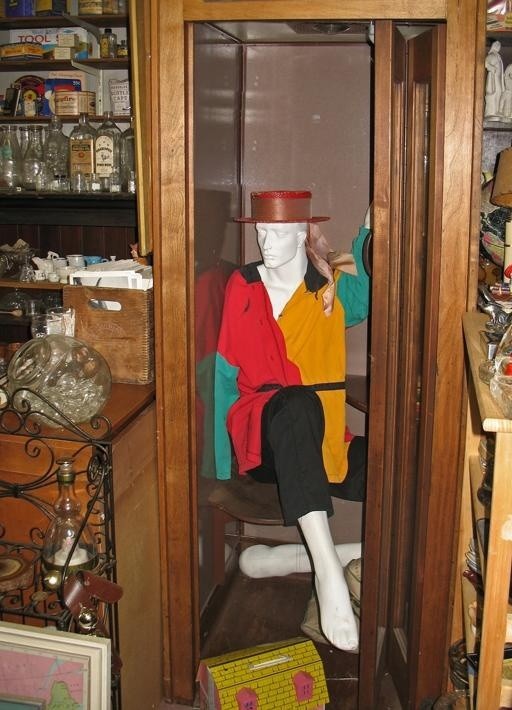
[231,190,330,224]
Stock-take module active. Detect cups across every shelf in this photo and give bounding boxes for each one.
[0,242,110,284]
[0,288,62,316]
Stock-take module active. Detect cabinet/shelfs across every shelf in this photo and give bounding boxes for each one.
[478,0,511,317]
[459,311,512,710]
[2,1,154,256]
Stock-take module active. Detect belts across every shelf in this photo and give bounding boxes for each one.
[60,569,122,677]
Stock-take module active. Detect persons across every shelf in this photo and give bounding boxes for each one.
[213,188,373,653]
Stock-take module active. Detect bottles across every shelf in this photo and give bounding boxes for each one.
[44,306,75,340]
[6,333,114,428]
[0,111,137,194]
[30,314,66,343]
[117,40,129,58]
[39,455,100,578]
[100,28,117,58]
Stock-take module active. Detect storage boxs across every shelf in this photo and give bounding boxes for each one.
[64,281,153,387]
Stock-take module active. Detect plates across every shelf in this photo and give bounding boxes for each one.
[464,536,482,575]
